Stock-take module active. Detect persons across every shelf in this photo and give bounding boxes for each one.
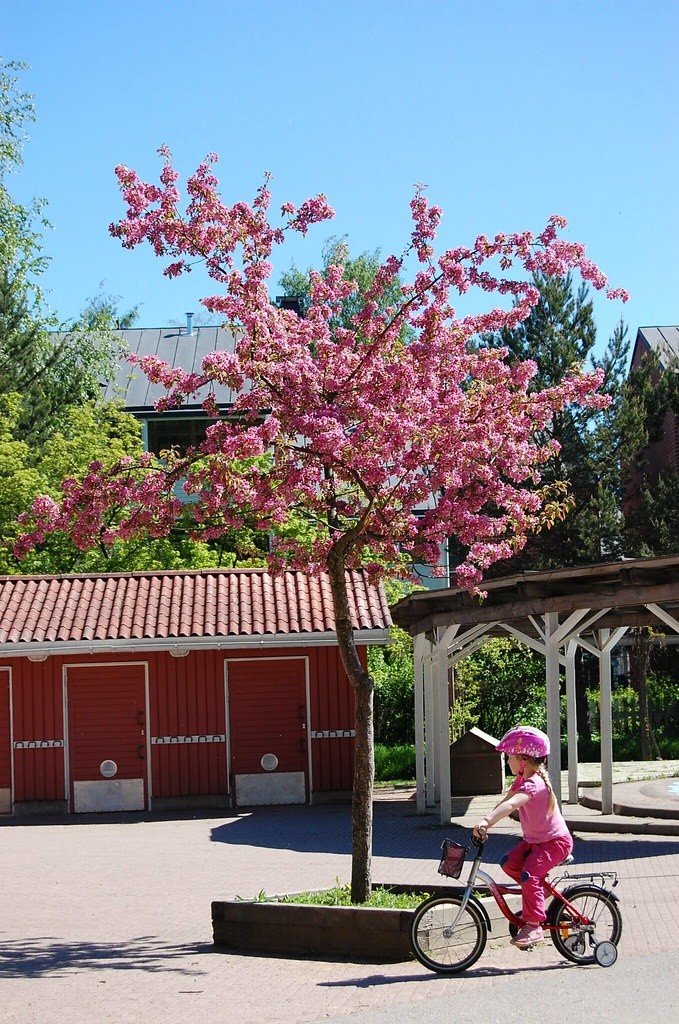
[472,726,574,948]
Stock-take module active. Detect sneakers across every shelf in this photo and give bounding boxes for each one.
[509,923,544,946]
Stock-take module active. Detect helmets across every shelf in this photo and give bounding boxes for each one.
[495,725,551,757]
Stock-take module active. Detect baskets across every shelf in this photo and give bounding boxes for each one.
[437,836,469,880]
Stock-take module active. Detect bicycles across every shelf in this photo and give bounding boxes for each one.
[407,825,623,974]
